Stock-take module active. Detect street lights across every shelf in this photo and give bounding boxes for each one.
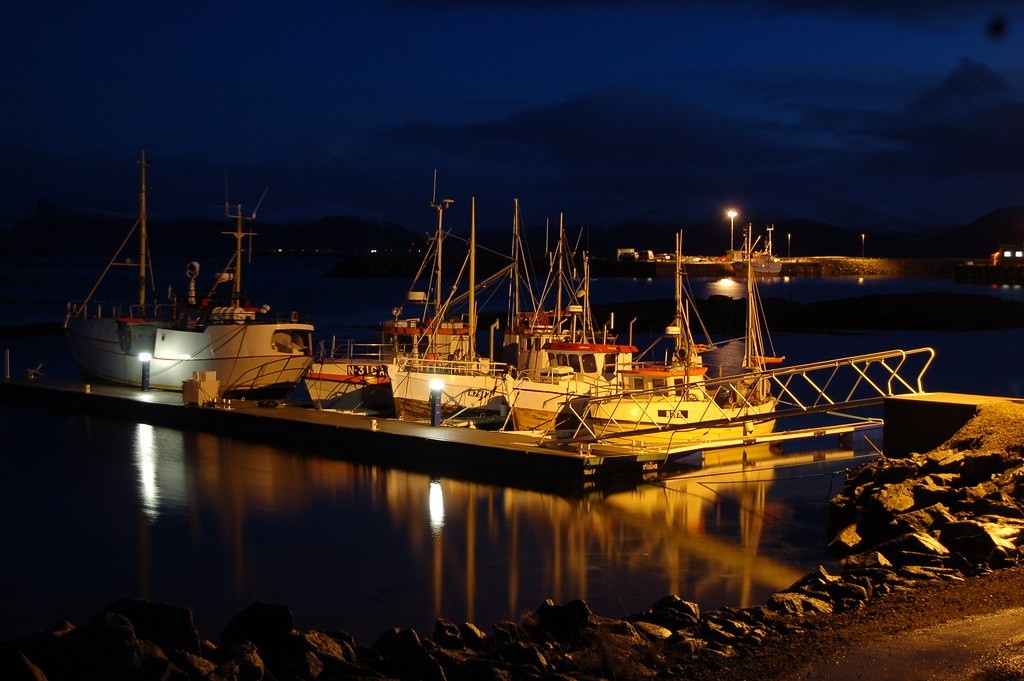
[721,207,741,254]
[860,233,866,256]
[787,234,792,256]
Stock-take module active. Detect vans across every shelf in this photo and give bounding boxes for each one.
[989,245,1024,271]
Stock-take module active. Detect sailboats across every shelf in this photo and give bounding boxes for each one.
[55,147,786,458]
